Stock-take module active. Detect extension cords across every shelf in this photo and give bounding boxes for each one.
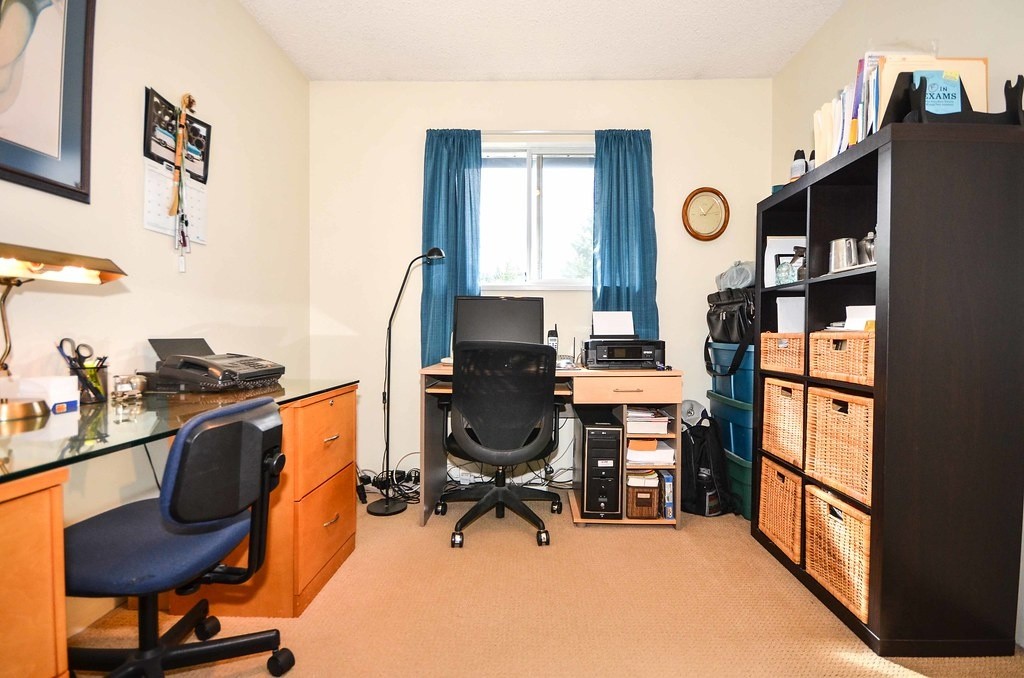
[523,485,549,491]
[363,478,420,494]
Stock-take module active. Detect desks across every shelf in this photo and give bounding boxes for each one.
[0,377,361,678]
[416,358,684,527]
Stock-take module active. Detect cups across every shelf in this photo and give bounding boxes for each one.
[129,375,147,392]
[114,375,133,392]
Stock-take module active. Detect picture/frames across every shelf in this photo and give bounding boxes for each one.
[0,0,96,205]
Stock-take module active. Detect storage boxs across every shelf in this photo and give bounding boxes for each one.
[656,469,674,519]
[702,341,756,520]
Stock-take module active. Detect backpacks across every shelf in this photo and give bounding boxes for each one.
[681,417,735,517]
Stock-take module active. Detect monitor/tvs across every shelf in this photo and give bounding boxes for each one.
[453,296,544,351]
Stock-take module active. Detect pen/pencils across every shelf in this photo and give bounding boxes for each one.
[95,356,107,372]
[57,346,105,401]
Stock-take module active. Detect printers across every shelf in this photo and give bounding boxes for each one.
[148,338,285,383]
[581,314,665,370]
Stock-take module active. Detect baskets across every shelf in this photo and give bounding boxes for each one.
[806,484,870,624]
[809,331,875,387]
[761,377,804,469]
[760,333,805,375]
[758,457,802,564]
[805,387,874,506]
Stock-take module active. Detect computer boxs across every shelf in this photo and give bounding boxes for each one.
[574,407,624,520]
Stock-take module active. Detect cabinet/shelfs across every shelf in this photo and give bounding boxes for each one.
[755,121,1024,659]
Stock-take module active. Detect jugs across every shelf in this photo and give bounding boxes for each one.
[829,238,858,273]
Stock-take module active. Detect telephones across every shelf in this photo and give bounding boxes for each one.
[548,323,558,355]
[158,352,285,391]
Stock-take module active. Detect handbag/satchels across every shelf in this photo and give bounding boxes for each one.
[704,286,756,377]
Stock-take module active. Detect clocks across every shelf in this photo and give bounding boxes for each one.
[682,187,730,242]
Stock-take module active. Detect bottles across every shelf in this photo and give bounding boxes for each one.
[860,221,878,264]
[776,263,795,285]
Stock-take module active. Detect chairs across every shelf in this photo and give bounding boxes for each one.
[63,397,299,678]
[431,338,563,548]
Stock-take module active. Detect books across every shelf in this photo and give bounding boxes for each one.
[627,439,674,465]
[814,51,989,168]
[626,468,659,486]
[627,406,675,433]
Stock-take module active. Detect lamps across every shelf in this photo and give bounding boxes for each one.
[0,240,128,437]
[366,248,446,517]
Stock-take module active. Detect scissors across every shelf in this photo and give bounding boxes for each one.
[60,338,92,368]
